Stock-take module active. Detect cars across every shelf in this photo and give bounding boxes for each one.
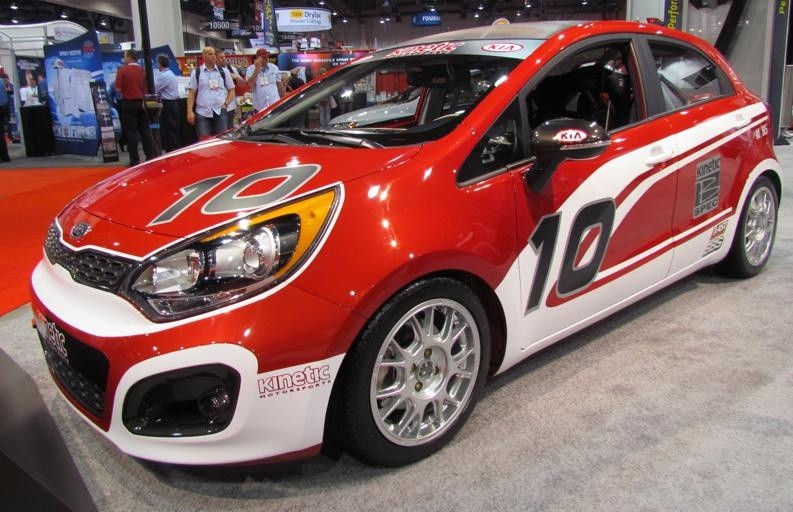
[28,20,783,470]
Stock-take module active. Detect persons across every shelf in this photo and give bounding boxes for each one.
[113,46,309,167]
[337,84,356,114]
[318,67,331,126]
[108,66,129,153]
[0,65,48,162]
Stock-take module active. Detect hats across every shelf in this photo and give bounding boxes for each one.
[255,48,271,58]
